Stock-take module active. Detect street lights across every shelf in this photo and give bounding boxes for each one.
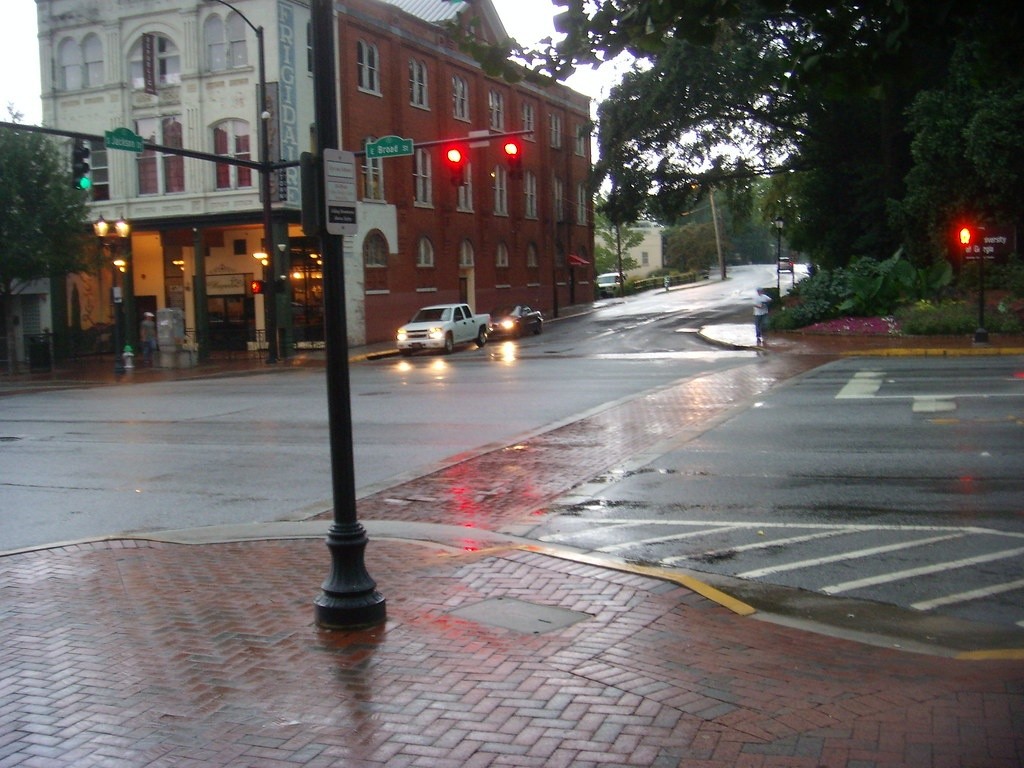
[92,213,130,375]
[775,215,786,296]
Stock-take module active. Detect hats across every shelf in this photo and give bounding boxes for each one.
[144,312,155,317]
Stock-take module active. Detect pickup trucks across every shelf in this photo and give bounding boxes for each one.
[396,303,492,354]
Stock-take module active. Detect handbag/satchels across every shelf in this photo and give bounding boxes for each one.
[151,340,157,349]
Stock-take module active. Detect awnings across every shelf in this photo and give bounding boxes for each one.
[568,255,591,267]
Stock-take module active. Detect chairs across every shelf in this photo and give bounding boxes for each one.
[63,328,104,364]
[28,341,51,371]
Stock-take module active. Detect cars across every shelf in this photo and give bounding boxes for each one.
[777,256,794,273]
[596,272,622,297]
[481,303,544,339]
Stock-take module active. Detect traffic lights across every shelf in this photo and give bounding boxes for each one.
[72,144,91,191]
[252,281,262,294]
[959,226,974,246]
[504,139,523,181]
[447,145,463,185]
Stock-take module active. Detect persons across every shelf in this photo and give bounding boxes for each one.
[140,311,158,367]
[752,288,772,343]
